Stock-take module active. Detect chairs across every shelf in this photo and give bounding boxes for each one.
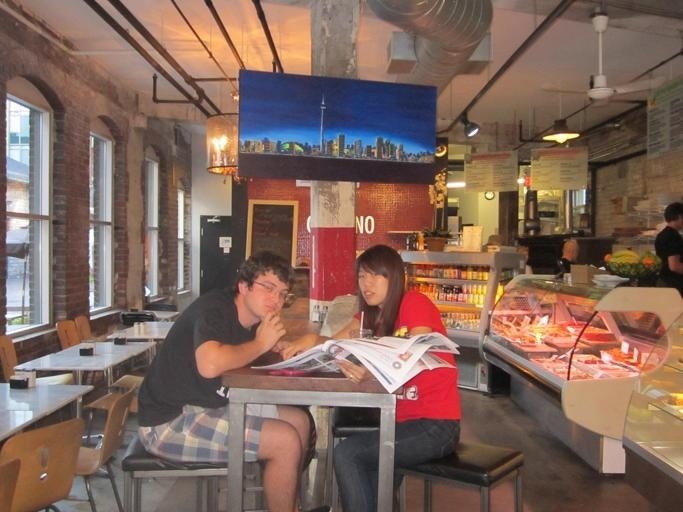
[0,305,185,511]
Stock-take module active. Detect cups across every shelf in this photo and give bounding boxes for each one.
[349,329,372,340]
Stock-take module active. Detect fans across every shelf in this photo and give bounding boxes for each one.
[536,12,665,102]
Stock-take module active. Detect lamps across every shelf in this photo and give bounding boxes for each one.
[540,83,581,144]
[459,114,484,138]
[203,90,254,186]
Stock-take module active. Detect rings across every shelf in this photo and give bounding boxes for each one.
[348,375,354,380]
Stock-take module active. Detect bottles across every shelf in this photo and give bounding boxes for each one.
[415,264,488,280]
[410,283,486,304]
[438,310,479,330]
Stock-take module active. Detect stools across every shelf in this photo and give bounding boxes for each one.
[123,423,230,511]
[397,443,525,512]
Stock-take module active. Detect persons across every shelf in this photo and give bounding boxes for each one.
[554,239,581,274]
[478,234,534,281]
[136,252,309,510]
[654,202,682,298]
[279,244,460,512]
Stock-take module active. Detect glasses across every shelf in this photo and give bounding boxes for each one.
[253,281,295,305]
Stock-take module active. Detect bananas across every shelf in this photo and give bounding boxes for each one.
[610,250,640,266]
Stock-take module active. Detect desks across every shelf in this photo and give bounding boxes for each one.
[223,290,396,511]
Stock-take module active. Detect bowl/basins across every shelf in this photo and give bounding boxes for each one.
[605,260,661,280]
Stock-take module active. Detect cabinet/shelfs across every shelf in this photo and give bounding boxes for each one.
[618,316,683,512]
[394,248,520,393]
[483,276,683,476]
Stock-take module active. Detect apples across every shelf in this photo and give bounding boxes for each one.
[642,256,656,268]
